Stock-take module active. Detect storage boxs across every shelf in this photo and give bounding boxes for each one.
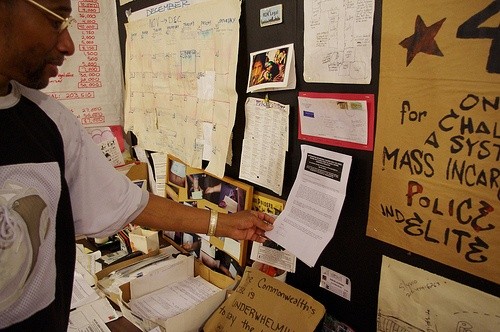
[121,162,148,180]
[93,227,239,332]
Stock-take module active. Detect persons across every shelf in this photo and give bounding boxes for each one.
[252,53,270,84]
[1,0,275,332]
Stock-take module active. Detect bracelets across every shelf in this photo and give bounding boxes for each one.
[207,207,219,235]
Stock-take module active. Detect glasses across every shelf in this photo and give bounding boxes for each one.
[28,0,74,34]
